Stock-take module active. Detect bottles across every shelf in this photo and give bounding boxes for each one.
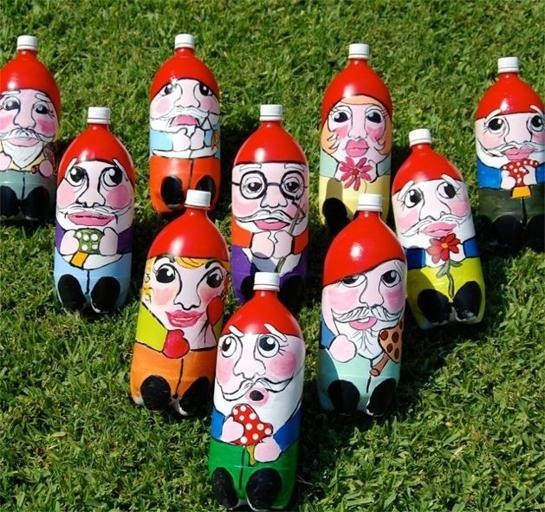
[317,42,394,226]
[205,271,306,512]
[390,127,486,331]
[230,101,313,309]
[0,32,62,220]
[129,187,231,417]
[52,104,138,320]
[475,55,545,259]
[147,33,221,214]
[315,192,409,419]
